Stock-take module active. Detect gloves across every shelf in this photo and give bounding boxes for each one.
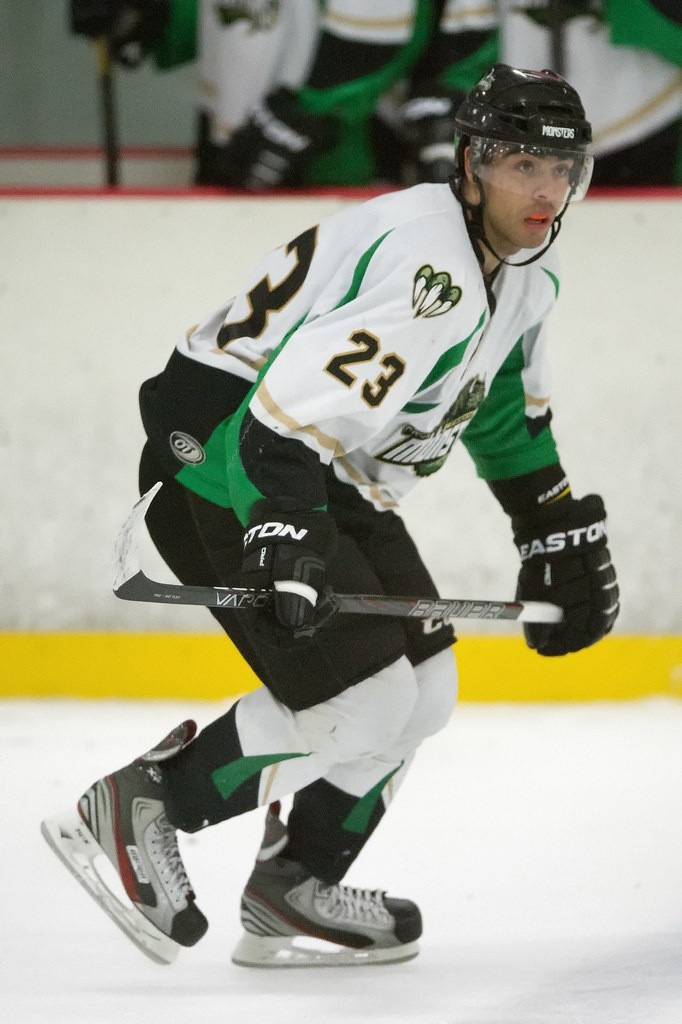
[237,501,340,648]
[402,96,461,187]
[222,89,330,193]
[513,494,620,657]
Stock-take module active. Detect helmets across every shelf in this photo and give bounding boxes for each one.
[452,64,592,190]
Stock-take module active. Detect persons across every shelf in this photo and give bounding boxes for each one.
[38,62,619,968]
[66,0,682,193]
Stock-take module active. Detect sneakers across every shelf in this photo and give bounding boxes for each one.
[41,716,210,964]
[231,796,424,969]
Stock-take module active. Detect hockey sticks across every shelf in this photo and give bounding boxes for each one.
[108,480,568,626]
